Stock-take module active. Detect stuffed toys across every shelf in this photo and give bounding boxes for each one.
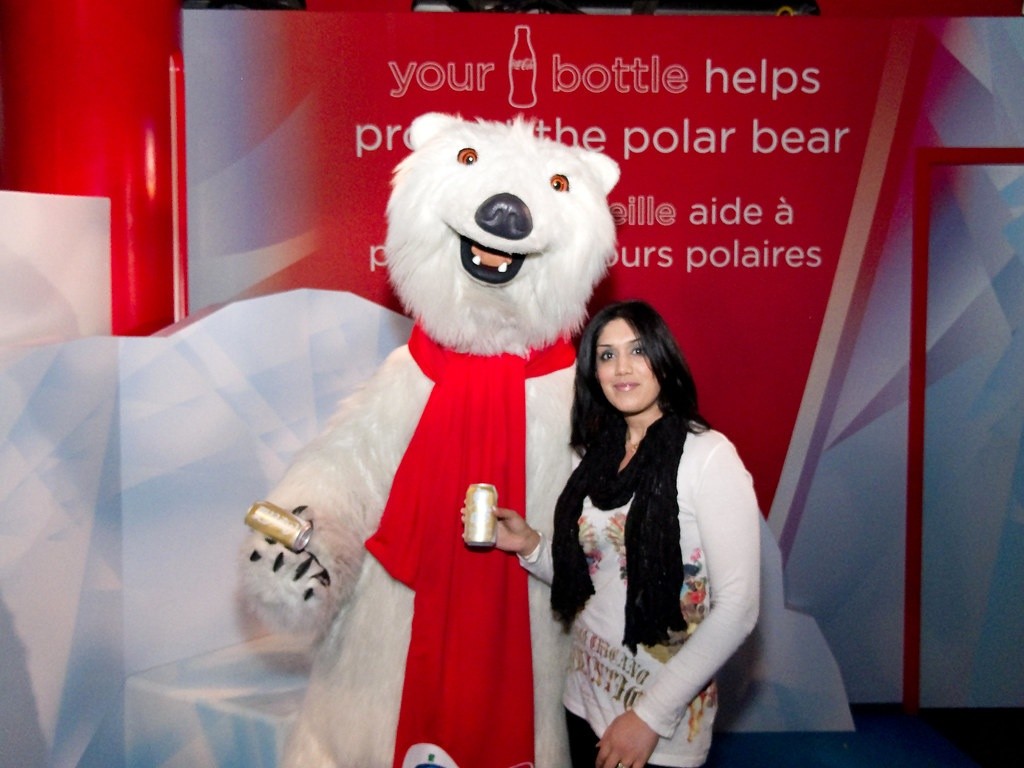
[237,112,621,768]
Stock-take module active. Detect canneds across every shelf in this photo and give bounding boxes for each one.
[244,501,312,551]
[465,483,498,547]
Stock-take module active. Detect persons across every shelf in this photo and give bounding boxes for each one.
[461,299,761,768]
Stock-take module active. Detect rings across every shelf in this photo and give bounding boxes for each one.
[617,761,624,768]
